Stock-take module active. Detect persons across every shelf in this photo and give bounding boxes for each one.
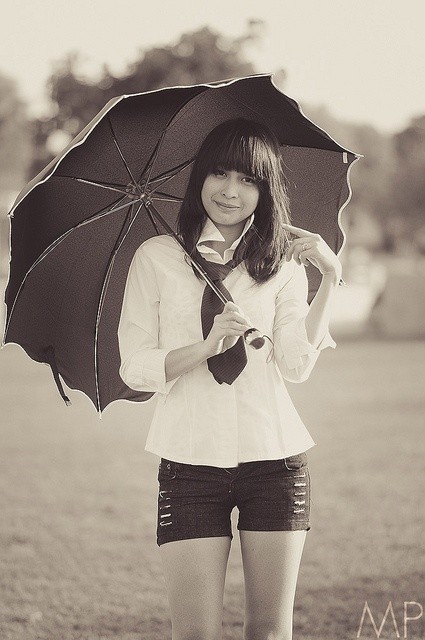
[116,118,344,640]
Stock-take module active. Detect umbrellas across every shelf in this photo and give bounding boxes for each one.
[1,72,365,423]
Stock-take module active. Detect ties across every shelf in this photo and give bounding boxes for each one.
[190,221,248,385]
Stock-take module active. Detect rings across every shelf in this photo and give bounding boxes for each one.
[301,243,308,251]
